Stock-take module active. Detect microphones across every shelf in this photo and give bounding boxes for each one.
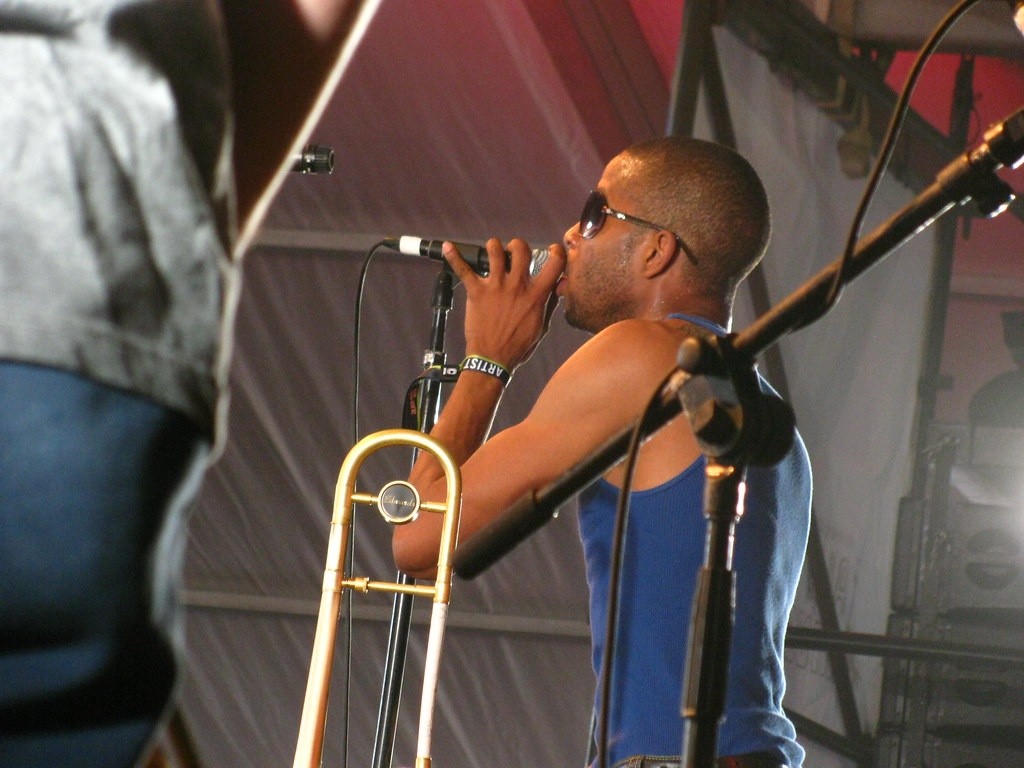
[381,234,564,285]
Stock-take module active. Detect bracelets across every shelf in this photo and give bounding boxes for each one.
[400,353,514,433]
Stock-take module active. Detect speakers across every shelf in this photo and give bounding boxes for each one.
[867,494,1023,767]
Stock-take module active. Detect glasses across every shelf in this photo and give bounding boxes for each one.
[577,189,699,267]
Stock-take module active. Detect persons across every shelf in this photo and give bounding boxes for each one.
[0,0,382,768]
[389,136,813,768]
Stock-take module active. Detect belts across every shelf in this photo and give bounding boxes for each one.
[611,750,783,768]
